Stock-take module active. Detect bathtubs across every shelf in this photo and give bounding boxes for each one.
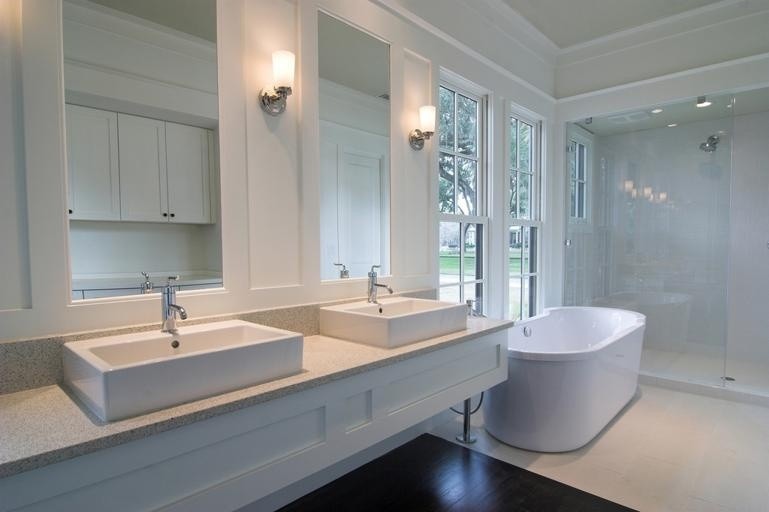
[483,306,648,453]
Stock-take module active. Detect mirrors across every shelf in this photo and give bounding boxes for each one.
[315,6,394,282]
[59,1,227,305]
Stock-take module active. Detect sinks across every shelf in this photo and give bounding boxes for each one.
[319,297,467,350]
[62,319,303,422]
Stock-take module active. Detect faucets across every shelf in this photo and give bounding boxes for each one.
[335,264,349,279]
[161,275,187,331]
[468,299,487,317]
[141,271,154,294]
[368,265,393,303]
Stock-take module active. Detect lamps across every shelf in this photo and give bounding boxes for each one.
[408,102,443,153]
[258,46,299,119]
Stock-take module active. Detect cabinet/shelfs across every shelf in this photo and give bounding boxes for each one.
[119,110,211,225]
[62,102,121,223]
